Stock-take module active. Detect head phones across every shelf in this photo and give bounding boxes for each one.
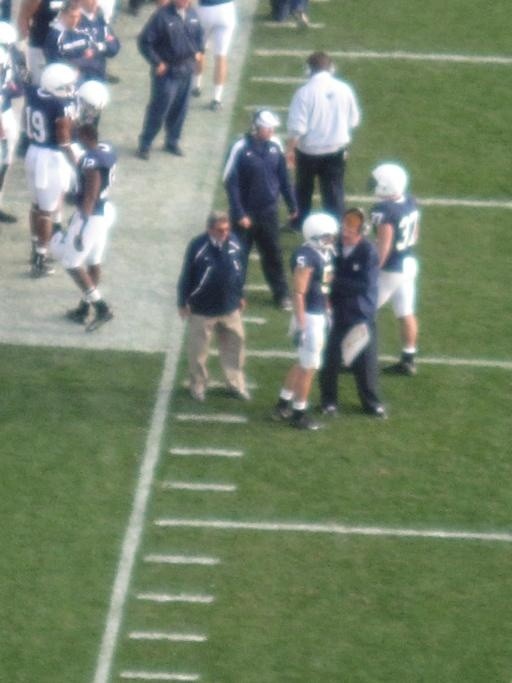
[303,52,336,75]
[249,108,268,136]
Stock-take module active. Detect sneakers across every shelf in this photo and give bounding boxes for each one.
[190,80,206,98]
[67,297,100,330]
[387,349,420,380]
[206,95,226,113]
[360,398,394,424]
[279,298,293,317]
[196,389,207,405]
[292,409,318,432]
[225,380,257,405]
[132,145,160,161]
[321,405,342,428]
[27,237,58,281]
[272,400,293,422]
[168,144,189,161]
[88,299,121,333]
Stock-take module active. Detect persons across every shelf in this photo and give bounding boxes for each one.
[190,0,238,112]
[361,163,421,379]
[268,213,341,431]
[137,0,206,160]
[222,109,299,311]
[176,211,251,401]
[312,207,388,420]
[285,52,361,235]
[269,0,308,26]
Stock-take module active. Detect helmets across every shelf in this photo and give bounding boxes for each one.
[251,107,282,135]
[369,162,410,199]
[300,210,342,246]
[79,79,115,113]
[38,63,80,97]
